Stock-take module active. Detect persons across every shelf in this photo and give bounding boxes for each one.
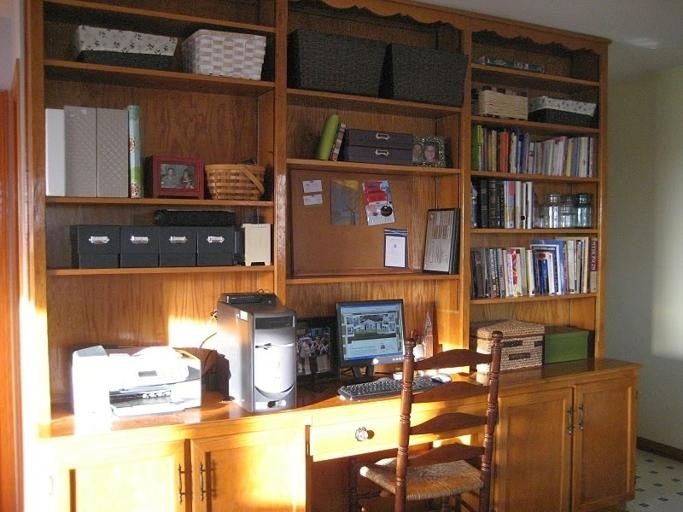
[295,328,328,374]
[412,142,422,161]
[421,143,438,162]
[160,167,178,188]
[178,168,195,189]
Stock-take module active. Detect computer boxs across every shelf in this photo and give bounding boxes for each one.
[215,301,297,414]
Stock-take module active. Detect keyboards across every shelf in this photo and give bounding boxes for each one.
[337,376,433,401]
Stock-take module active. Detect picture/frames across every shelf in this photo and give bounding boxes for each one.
[412,134,450,168]
[417,206,461,275]
[291,314,342,390]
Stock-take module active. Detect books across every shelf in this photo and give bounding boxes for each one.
[477,55,544,73]
[328,123,346,161]
[469,124,597,300]
[125,103,144,199]
[313,113,339,161]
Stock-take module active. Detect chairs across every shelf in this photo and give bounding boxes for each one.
[346,329,507,512]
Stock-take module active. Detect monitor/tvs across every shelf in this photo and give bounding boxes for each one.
[335,299,407,384]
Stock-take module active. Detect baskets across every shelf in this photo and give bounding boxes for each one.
[205,162,268,200]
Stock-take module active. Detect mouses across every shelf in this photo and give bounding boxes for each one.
[431,374,452,383]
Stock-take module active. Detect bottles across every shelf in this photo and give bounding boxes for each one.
[422,311,434,358]
[540,190,594,229]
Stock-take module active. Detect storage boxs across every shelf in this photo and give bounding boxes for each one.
[468,318,545,373]
[77,21,180,71]
[179,27,268,80]
[341,126,417,166]
[471,84,531,122]
[529,95,597,126]
[545,324,591,363]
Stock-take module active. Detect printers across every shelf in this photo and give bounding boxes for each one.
[72,344,202,418]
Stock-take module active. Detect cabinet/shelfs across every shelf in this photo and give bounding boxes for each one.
[23,1,617,424]
[310,396,491,461]
[431,356,642,512]
[37,422,309,511]
